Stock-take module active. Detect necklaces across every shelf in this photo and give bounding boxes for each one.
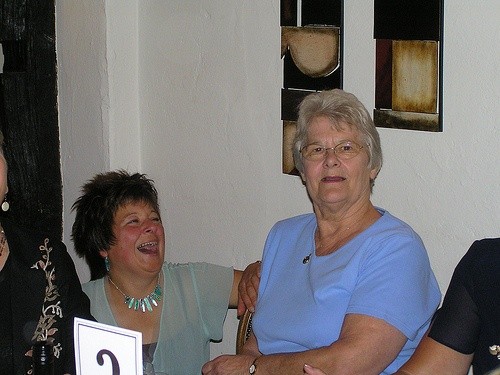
[303,217,358,264]
[0,227,7,257]
[108,273,163,313]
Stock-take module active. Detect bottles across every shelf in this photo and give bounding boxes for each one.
[31,341,54,375]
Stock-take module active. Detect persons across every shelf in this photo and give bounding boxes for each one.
[303,237,500,375]
[71,169,262,375]
[201,89,441,375]
[0,147,99,375]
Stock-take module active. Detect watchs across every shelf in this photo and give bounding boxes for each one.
[249,358,259,375]
[256,261,262,266]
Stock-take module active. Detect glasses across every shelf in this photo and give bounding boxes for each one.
[299,141,370,161]
